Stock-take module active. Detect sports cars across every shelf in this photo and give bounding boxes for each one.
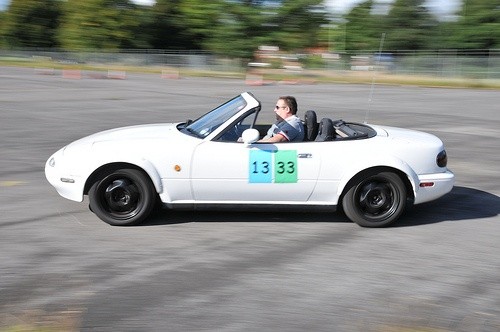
[42,91,454,227]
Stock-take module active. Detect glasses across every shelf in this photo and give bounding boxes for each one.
[276,106,287,110]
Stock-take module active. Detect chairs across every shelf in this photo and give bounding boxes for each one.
[304,110,335,141]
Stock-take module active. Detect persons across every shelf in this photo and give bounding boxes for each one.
[237,96,305,142]
